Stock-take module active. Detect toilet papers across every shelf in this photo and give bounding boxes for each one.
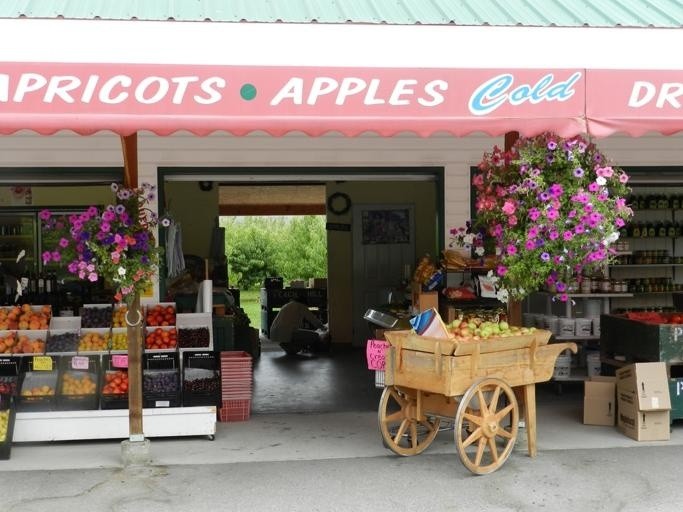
[195,279,213,314]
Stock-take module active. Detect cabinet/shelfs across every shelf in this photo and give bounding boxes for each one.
[1,204,104,315]
[598,178,683,315]
[553,335,602,384]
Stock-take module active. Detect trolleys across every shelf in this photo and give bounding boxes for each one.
[377,329,578,475]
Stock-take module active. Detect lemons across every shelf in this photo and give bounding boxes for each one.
[112,333,128,349]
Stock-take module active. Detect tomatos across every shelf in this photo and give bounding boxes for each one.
[103,370,129,399]
[668,313,683,324]
[145,305,178,325]
[146,327,178,348]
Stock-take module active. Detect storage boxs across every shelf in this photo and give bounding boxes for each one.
[581,361,672,442]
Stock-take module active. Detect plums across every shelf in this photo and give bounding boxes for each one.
[81,306,113,327]
[46,332,78,351]
[143,372,179,392]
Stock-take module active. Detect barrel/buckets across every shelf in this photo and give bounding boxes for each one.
[587,353,602,377]
[522,312,600,338]
[551,354,571,378]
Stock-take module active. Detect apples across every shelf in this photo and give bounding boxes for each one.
[1,409,10,442]
[0,376,17,393]
[21,385,55,400]
[0,332,45,354]
[448,319,537,339]
[0,304,52,329]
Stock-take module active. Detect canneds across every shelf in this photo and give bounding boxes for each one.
[610,242,634,266]
[635,249,682,265]
[581,276,682,294]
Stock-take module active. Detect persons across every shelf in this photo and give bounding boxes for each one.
[269,295,327,356]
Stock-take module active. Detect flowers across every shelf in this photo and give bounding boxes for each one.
[35,182,172,311]
[448,134,634,305]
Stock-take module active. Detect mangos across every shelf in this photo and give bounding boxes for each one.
[62,373,96,400]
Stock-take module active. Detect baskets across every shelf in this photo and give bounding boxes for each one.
[0,397,16,460]
[218,350,253,424]
[0,351,223,412]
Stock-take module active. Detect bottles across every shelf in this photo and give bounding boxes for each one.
[18,269,57,294]
[0,224,25,236]
[424,266,444,293]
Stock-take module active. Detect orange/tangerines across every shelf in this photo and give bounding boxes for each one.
[111,306,137,327]
